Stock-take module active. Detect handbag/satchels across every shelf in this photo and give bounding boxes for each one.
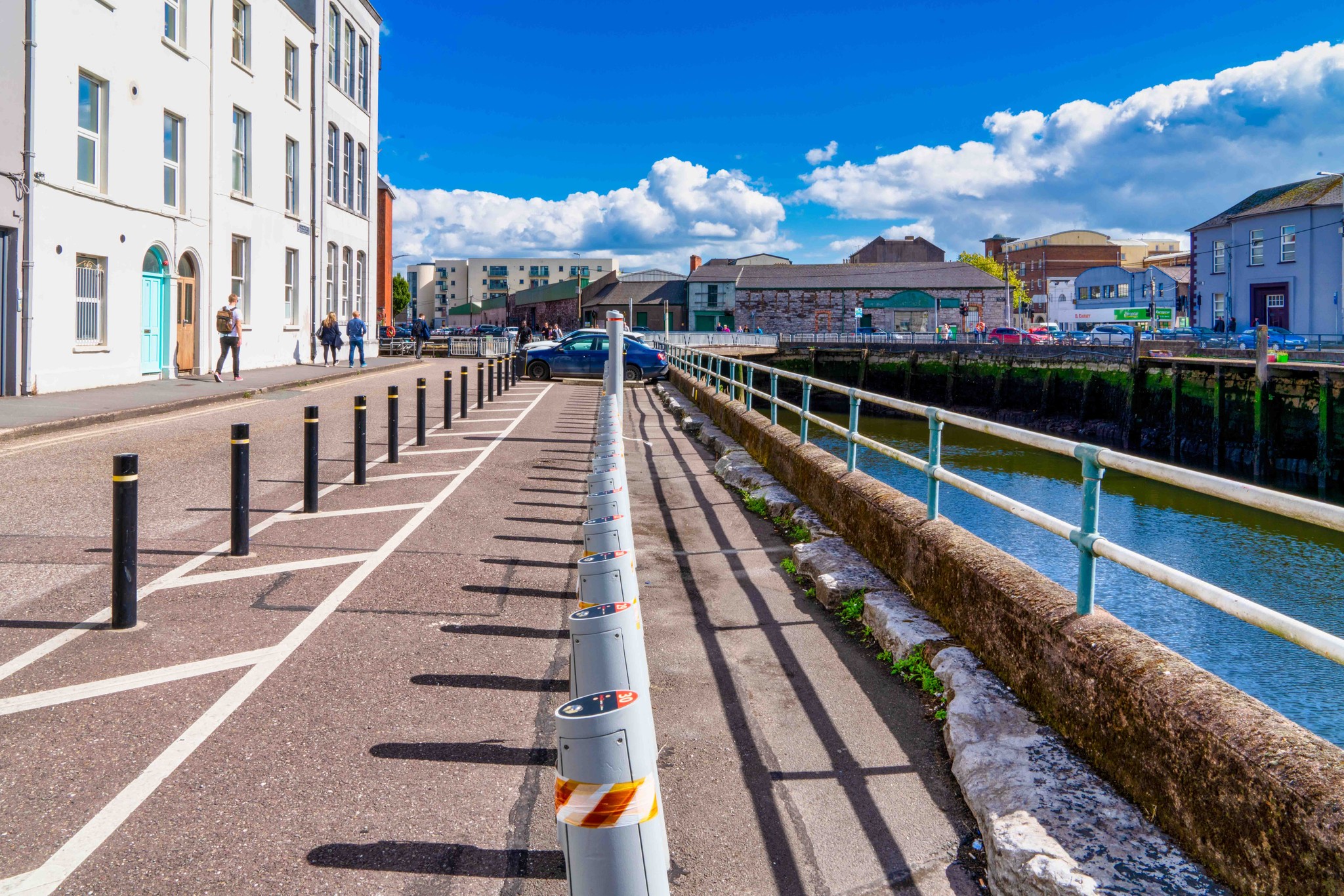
[316,321,323,340]
[939,327,942,335]
[423,322,431,340]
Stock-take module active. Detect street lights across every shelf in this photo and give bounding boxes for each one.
[573,252,581,328]
[504,275,510,326]
[467,287,472,327]
[444,290,449,327]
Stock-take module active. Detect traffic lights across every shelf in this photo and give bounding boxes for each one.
[963,306,968,315]
[1175,296,1181,312]
[959,303,964,314]
[1146,307,1151,317]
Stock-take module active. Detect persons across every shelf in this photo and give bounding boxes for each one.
[940,323,953,343]
[716,322,730,332]
[515,320,533,349]
[1214,316,1225,332]
[541,322,563,341]
[623,322,630,332]
[874,329,878,333]
[754,326,763,334]
[981,323,988,343]
[214,294,243,383]
[410,313,429,360]
[736,324,750,333]
[1227,317,1237,333]
[400,325,402,328]
[346,311,367,368]
[321,311,342,367]
[974,325,979,344]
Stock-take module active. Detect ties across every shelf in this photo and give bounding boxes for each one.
[549,331,552,341]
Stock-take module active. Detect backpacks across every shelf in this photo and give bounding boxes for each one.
[541,326,545,336]
[412,320,422,335]
[216,306,238,336]
[973,329,978,335]
[553,331,557,339]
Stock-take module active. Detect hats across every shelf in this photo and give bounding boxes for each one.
[536,324,538,325]
[1255,316,1260,318]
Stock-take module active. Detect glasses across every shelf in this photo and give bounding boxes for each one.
[235,300,238,302]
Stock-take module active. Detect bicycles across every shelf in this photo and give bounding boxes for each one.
[397,336,419,355]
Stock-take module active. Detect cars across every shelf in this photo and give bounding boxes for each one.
[1146,327,1235,348]
[1062,331,1090,346]
[1238,326,1308,350]
[1049,331,1066,345]
[1027,327,1053,345]
[509,336,669,385]
[1028,309,1034,319]
[1022,302,1028,314]
[987,326,1042,344]
[424,325,521,337]
[625,326,651,334]
[379,325,409,337]
[520,329,651,350]
[854,326,903,343]
[1089,325,1153,347]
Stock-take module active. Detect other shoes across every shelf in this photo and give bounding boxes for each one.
[349,365,354,368]
[324,362,329,367]
[214,373,224,383]
[333,360,339,366]
[360,363,367,367]
[416,356,422,359]
[234,376,243,381]
